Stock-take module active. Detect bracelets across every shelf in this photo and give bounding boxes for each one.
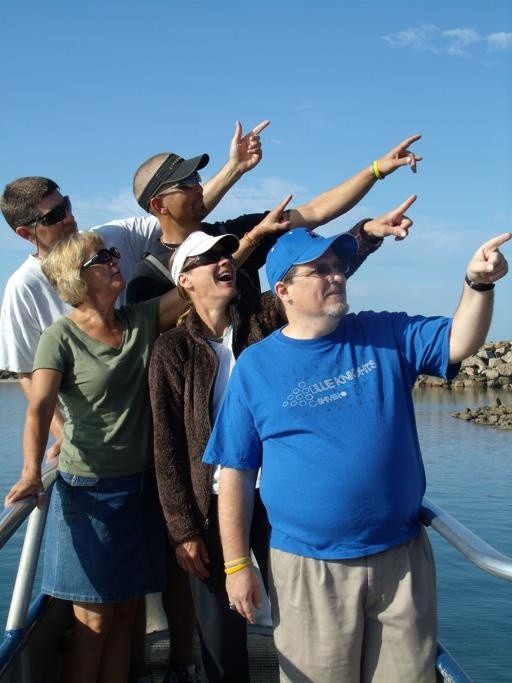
[370,158,384,181]
[243,232,261,248]
[465,275,496,291]
[223,556,252,574]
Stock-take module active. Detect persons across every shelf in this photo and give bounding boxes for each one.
[125,132,423,683]
[203,227,511,683]
[0,119,271,682]
[4,193,292,682]
[149,194,416,683]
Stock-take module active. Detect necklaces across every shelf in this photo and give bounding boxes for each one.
[161,239,177,250]
[205,321,232,341]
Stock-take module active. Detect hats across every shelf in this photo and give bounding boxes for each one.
[171,230,241,287]
[265,227,359,294]
[138,154,209,213]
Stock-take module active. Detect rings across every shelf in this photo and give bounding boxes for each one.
[228,601,236,611]
[39,491,46,496]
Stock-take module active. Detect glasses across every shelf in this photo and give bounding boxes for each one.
[181,251,234,273]
[156,173,201,197]
[82,247,121,270]
[285,261,349,281]
[23,196,71,226]
